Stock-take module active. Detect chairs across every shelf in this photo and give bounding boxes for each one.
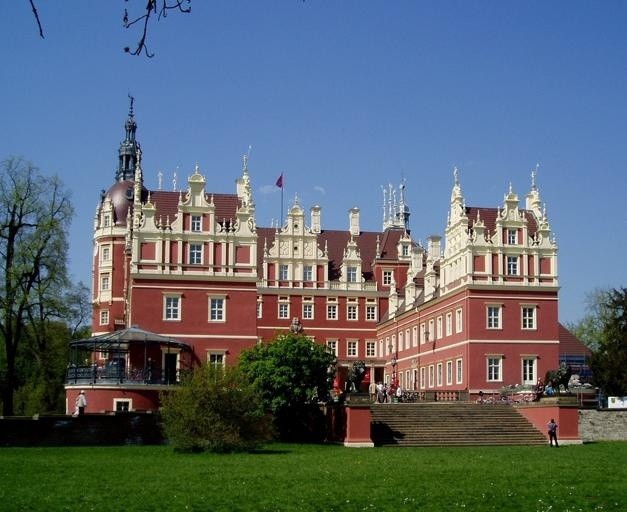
[396,391,417,402]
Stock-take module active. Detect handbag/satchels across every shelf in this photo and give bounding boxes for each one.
[547,430,553,435]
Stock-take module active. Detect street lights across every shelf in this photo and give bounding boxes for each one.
[391,356,396,401]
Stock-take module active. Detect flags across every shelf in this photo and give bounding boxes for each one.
[276,175,283,188]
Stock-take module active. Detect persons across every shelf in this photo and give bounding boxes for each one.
[148,357,156,380]
[547,419,559,447]
[76,390,88,415]
[74,393,81,416]
[536,377,556,396]
[369,381,402,403]
